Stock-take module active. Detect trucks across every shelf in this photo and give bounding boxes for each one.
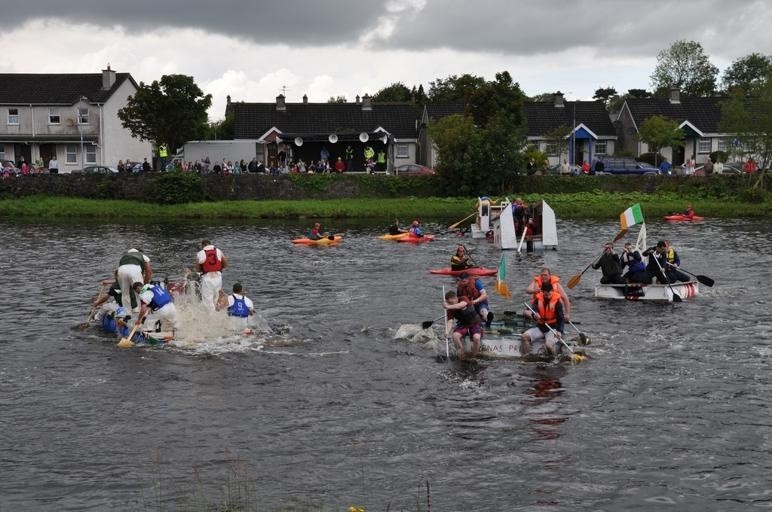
[169,138,268,176]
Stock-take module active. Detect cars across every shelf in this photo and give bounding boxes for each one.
[727,162,772,172]
[0,159,21,177]
[123,161,145,172]
[548,162,591,176]
[391,164,437,177]
[692,165,743,175]
[70,164,120,175]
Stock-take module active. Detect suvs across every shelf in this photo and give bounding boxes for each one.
[760,150,772,169]
[594,154,665,182]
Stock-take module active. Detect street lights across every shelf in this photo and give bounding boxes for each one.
[79,96,88,169]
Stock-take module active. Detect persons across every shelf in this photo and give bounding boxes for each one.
[408,220,423,236]
[413,269,571,359]
[309,222,327,241]
[450,246,474,271]
[512,196,539,237]
[92,239,255,353]
[389,218,408,235]
[682,203,695,220]
[521,136,772,178]
[592,239,691,284]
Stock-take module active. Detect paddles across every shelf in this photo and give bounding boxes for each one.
[118,308,151,346]
[325,235,334,240]
[666,262,714,287]
[568,320,587,346]
[448,212,477,229]
[568,229,629,289]
[652,253,683,302]
[423,314,445,329]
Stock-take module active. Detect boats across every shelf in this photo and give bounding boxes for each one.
[97,283,265,356]
[375,231,413,239]
[495,197,560,251]
[593,278,699,303]
[397,233,436,245]
[461,311,548,360]
[289,235,343,245]
[469,195,512,240]
[428,265,499,277]
[664,214,704,223]
